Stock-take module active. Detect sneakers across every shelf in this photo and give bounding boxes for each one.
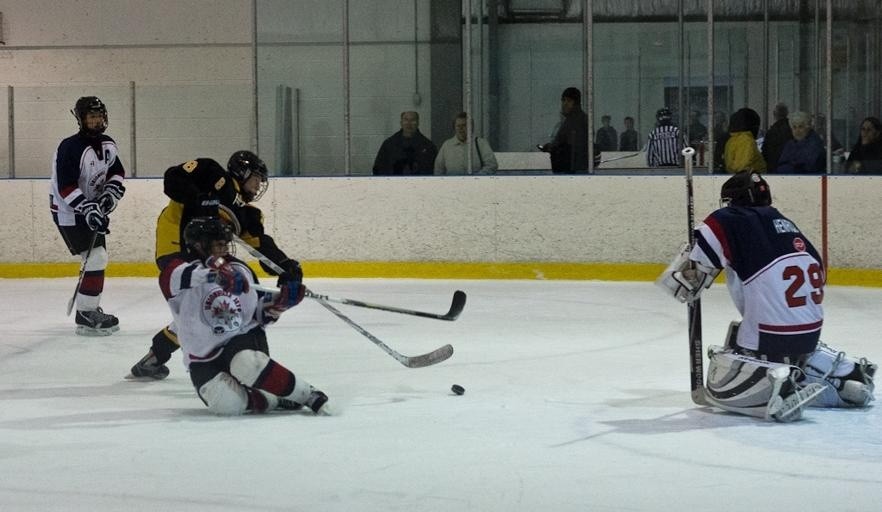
[839,362,878,406]
[75,309,113,329]
[304,390,329,414]
[763,363,806,423]
[276,395,303,410]
[131,353,170,380]
[102,313,119,326]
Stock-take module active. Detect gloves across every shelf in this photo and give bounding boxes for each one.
[217,203,241,237]
[96,180,126,216]
[205,255,249,296]
[277,258,304,288]
[264,281,307,316]
[74,199,110,235]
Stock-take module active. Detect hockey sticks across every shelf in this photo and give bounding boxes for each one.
[681,147,714,404]
[233,236,454,370]
[65,206,106,315]
[245,283,468,321]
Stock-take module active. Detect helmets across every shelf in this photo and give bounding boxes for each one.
[183,213,236,266]
[69,96,108,138]
[718,169,772,210]
[655,108,672,126]
[227,150,269,204]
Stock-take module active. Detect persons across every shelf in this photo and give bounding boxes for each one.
[775,111,829,173]
[680,110,709,145]
[811,114,847,151]
[654,174,877,423]
[132,149,303,382]
[372,111,439,175]
[643,108,689,167]
[156,216,335,413]
[433,112,499,176]
[594,115,618,151]
[846,117,882,176]
[620,117,640,151]
[715,111,731,175]
[762,102,796,172]
[48,95,127,338]
[724,107,768,174]
[536,87,600,176]
